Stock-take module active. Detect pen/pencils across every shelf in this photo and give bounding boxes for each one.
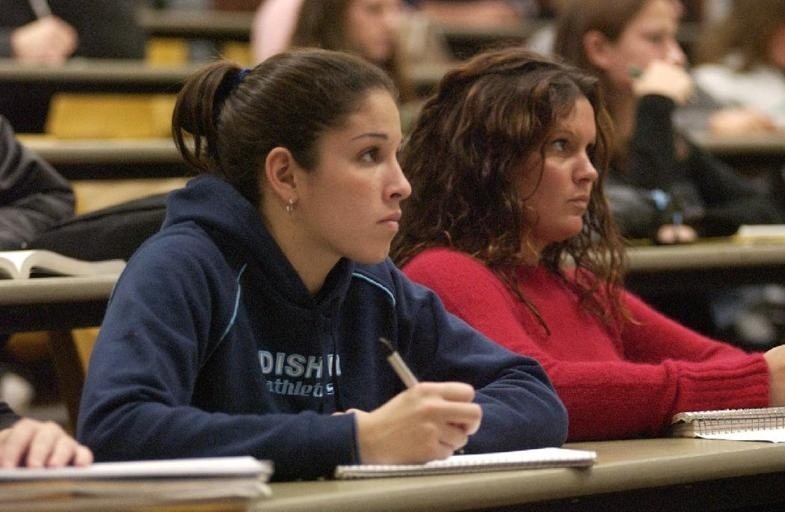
[30,1,54,19]
[377,338,470,455]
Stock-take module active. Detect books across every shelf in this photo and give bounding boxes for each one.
[0,249,127,279]
[335,447,596,479]
[0,455,274,512]
[671,407,785,438]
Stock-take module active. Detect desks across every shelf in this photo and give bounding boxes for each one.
[0,0,785,512]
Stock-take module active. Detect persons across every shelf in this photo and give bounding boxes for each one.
[671,2,784,148]
[0,115,75,250]
[76,48,569,482]
[0,1,143,133]
[252,0,462,133]
[553,0,753,244]
[0,417,94,467]
[389,49,785,443]
